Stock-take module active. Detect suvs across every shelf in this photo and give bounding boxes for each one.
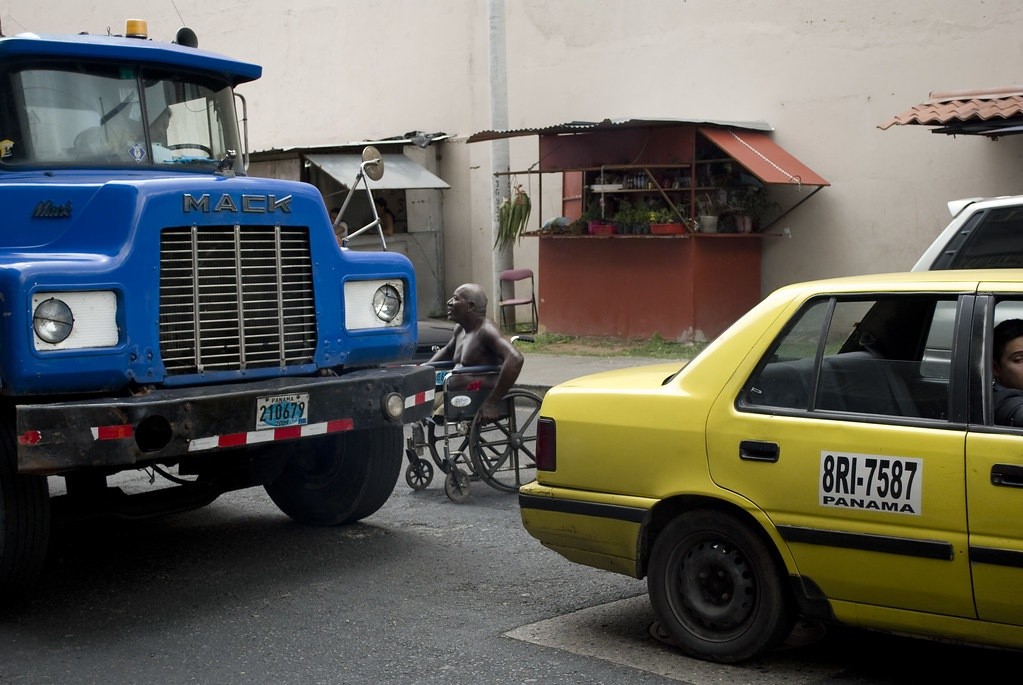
[910,194,1023,378]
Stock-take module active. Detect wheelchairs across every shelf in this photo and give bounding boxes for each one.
[406,335,544,502]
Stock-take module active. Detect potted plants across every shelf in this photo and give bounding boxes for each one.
[568,219,588,236]
[617,199,687,234]
[698,194,762,233]
[582,205,615,234]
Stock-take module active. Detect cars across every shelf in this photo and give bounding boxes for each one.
[518,267,1023,659]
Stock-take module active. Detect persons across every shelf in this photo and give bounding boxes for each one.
[428,284,524,426]
[329,210,348,247]
[74,106,172,165]
[993,318,1023,430]
[366,197,396,236]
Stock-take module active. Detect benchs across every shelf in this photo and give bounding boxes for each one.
[746,352,919,417]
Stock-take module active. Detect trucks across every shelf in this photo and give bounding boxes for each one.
[2,19,436,528]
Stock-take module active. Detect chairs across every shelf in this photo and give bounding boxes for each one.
[498,269,538,335]
